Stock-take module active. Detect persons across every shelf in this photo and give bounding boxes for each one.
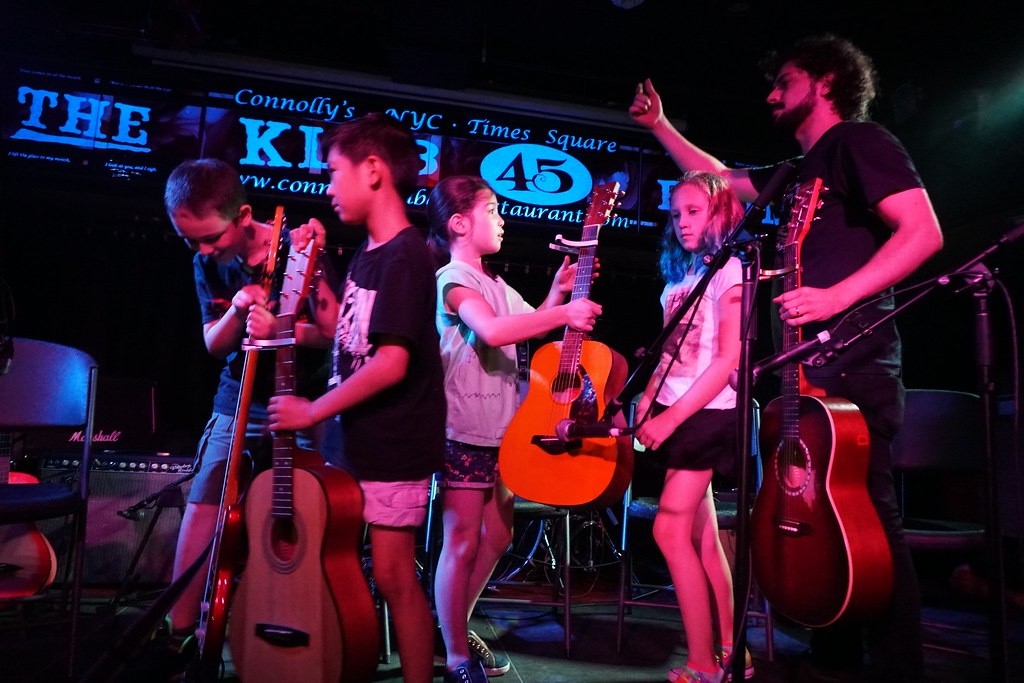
[115,113,604,683]
[628,33,945,683]
[610,166,755,683]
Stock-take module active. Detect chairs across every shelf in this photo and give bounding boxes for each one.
[613,390,775,662]
[0,335,99,683]
[888,381,1012,683]
[422,472,573,658]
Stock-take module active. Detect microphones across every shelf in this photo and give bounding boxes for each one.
[728,331,835,392]
[116,508,146,521]
[556,419,636,442]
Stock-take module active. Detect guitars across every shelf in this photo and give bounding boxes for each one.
[749,175,897,633]
[496,179,636,512]
[227,230,383,683]
[190,204,289,683]
[0,274,59,603]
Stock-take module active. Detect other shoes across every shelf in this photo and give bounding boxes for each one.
[671,665,729,683]
[667,645,755,681]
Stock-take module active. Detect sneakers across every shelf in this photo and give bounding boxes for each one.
[432,624,511,676]
[442,657,491,683]
[112,615,198,683]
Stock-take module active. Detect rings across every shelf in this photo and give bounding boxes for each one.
[795,306,801,317]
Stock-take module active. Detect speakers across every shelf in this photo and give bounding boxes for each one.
[33,451,199,597]
